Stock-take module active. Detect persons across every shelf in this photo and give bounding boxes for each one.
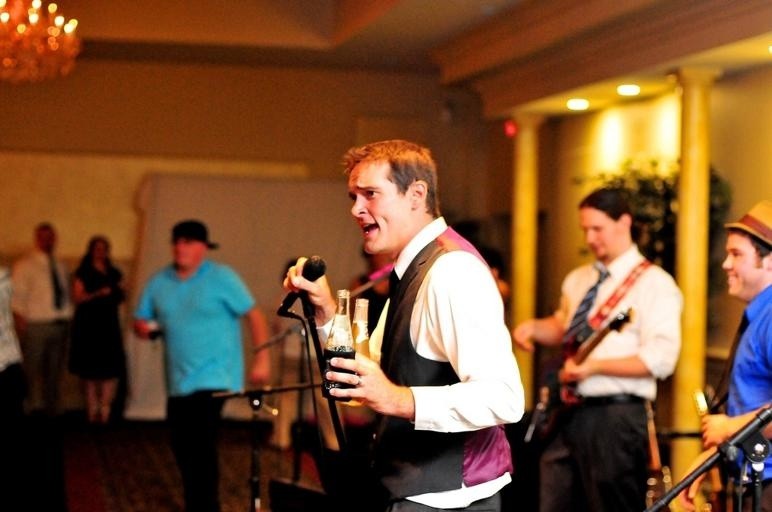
[677,197,768,511]
[285,141,527,512]
[131,220,271,508]
[284,242,396,438]
[511,186,683,511]
[0,218,129,507]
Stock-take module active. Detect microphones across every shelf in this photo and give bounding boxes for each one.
[276,254,327,317]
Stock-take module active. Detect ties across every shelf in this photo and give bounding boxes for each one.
[708,309,749,414]
[49,253,63,310]
[555,270,609,362]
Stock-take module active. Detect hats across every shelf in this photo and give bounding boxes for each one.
[724,200,772,252]
[172,220,221,249]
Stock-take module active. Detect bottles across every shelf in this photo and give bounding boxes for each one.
[321,288,370,408]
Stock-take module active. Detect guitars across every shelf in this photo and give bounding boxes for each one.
[520,310,635,456]
